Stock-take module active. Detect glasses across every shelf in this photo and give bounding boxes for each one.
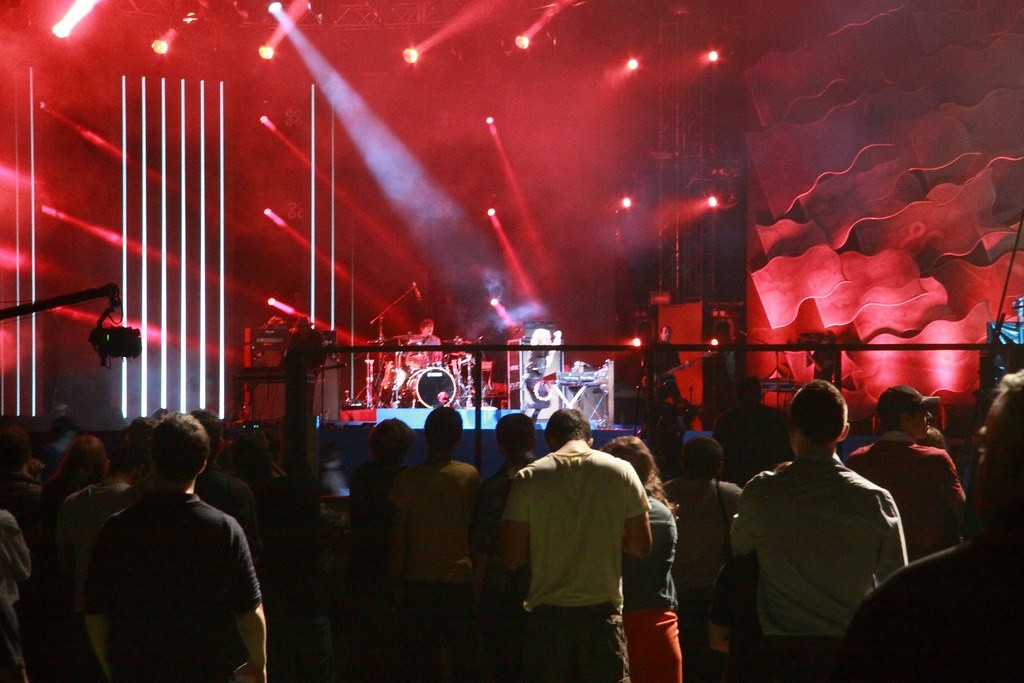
[974,427,1003,454]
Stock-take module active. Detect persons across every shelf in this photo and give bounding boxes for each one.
[0,407,687,683]
[806,331,843,390]
[652,323,685,405]
[525,329,563,421]
[407,318,443,363]
[671,371,1023,683]
[281,315,327,418]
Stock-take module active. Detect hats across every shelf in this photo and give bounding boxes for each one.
[874,385,941,418]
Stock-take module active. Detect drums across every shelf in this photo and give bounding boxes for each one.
[409,352,435,373]
[406,367,456,409]
[382,361,410,392]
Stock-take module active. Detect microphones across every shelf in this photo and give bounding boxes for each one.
[413,282,422,301]
[443,397,451,400]
[738,330,748,336]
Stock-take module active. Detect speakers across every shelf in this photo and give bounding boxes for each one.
[507,338,564,410]
[244,326,340,423]
[658,300,747,432]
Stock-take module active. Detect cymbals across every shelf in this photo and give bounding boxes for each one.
[443,340,473,344]
[394,334,423,339]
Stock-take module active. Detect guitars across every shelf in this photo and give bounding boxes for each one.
[647,351,720,393]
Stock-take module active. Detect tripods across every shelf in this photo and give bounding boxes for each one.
[355,349,384,410]
[452,355,476,409]
